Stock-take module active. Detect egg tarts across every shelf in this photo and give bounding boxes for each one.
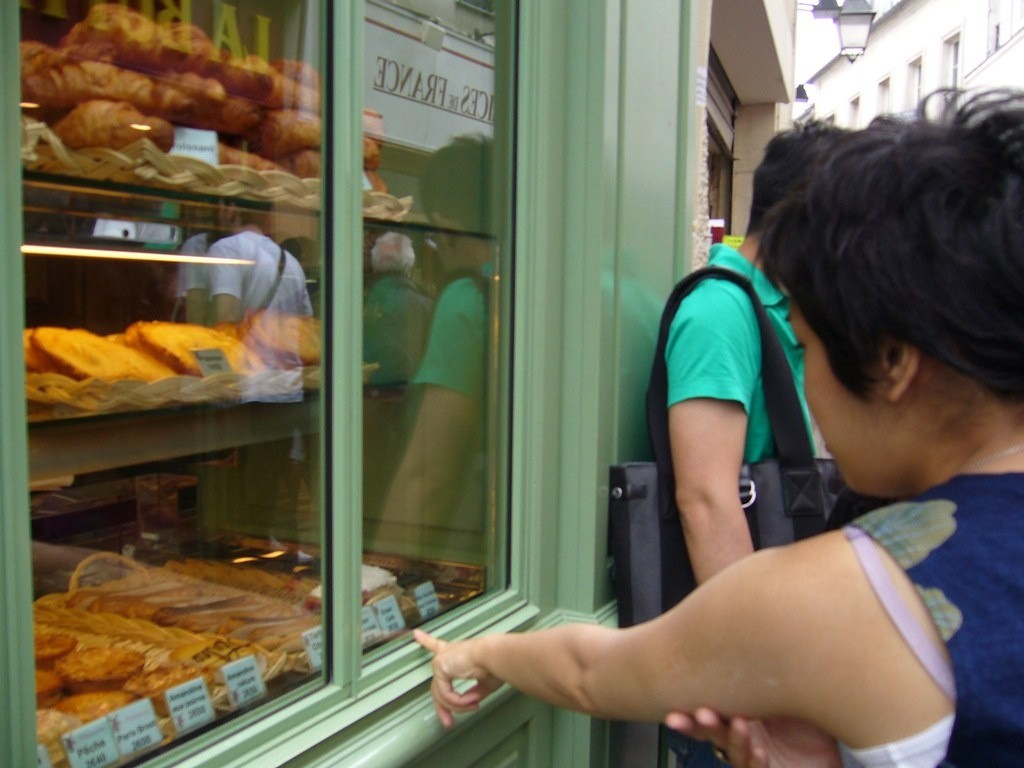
[34,631,216,756]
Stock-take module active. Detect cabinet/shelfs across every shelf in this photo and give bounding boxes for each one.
[15,170,501,768]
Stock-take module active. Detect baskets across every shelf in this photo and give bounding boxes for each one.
[33,552,403,766]
[24,360,380,412]
[21,114,413,222]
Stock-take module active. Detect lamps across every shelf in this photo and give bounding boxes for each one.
[810,0,842,19]
[833,0,876,64]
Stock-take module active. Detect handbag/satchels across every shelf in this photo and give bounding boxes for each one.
[609,268,900,629]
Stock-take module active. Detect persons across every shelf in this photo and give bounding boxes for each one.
[656,115,869,768]
[410,77,1024,768]
[147,163,326,560]
[364,128,494,603]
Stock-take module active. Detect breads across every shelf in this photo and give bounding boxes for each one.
[68,578,315,644]
[19,0,386,195]
[22,309,320,384]
